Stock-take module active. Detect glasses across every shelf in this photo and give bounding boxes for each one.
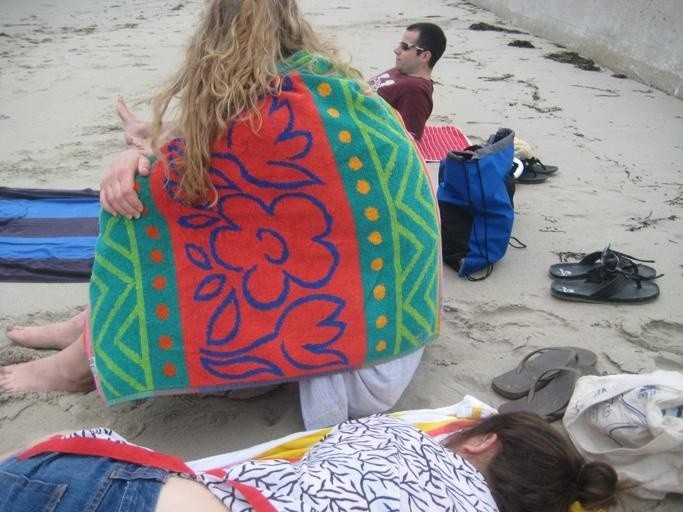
[601,243,663,280]
[399,41,425,51]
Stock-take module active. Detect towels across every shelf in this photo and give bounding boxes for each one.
[80,49,445,406]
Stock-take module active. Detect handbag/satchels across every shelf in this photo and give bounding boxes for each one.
[437,129,526,281]
[562,370,683,500]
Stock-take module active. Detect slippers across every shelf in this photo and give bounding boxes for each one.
[517,156,559,183]
[491,346,600,423]
[548,251,659,304]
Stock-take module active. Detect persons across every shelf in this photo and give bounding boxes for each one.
[1,1,444,433]
[0,409,624,510]
[114,23,448,151]
